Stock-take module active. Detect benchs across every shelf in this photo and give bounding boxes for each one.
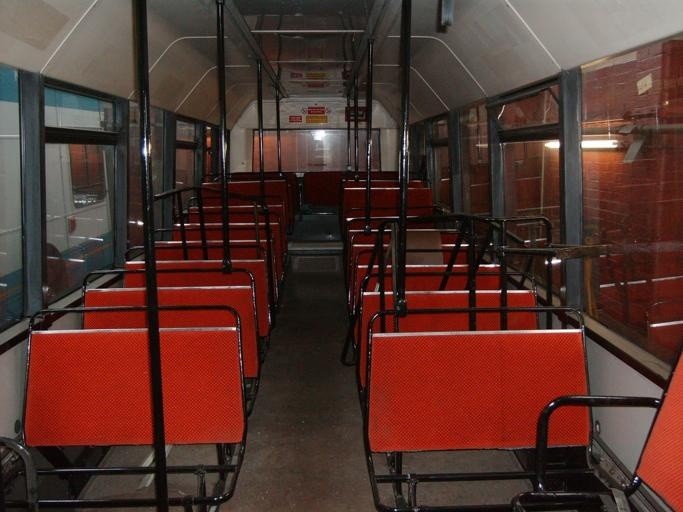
[0,168,304,511]
[330,169,681,512]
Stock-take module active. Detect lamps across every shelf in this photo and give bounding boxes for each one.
[541,133,626,150]
